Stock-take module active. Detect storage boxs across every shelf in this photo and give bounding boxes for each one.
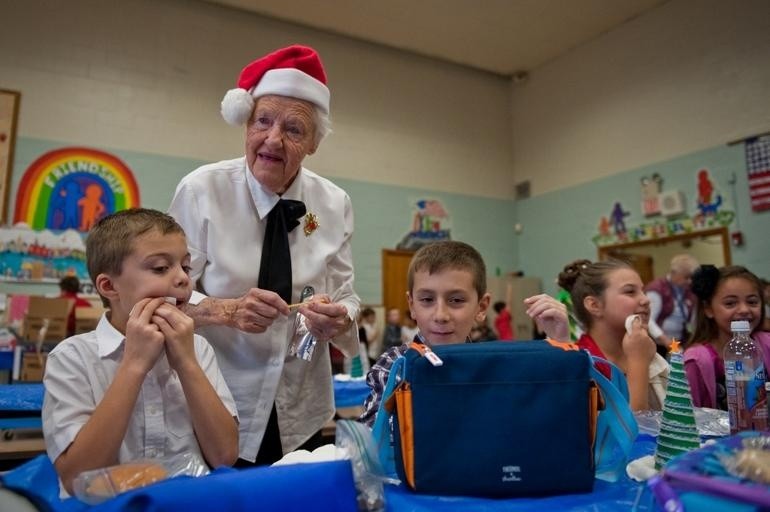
[0,293,113,384]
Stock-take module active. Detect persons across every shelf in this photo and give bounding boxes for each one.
[40,208,240,502]
[493,283,516,342]
[556,252,669,415]
[470,315,499,343]
[760,276,770,333]
[362,307,378,366]
[381,306,403,352]
[358,240,571,447]
[55,275,91,307]
[644,255,699,358]
[400,308,420,343]
[683,263,769,411]
[164,93,361,469]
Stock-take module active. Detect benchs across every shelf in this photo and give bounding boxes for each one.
[1,416,43,437]
[0,437,48,460]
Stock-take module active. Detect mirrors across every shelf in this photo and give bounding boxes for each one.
[596,227,730,289]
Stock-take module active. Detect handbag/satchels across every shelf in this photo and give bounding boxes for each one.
[387,343,600,500]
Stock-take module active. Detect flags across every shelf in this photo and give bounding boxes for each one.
[744,138,769,212]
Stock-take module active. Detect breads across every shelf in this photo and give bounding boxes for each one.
[85,463,164,497]
[738,446,769,482]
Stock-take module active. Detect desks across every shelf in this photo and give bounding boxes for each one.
[0,433,769,512]
[0,376,377,410]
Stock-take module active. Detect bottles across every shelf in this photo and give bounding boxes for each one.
[721,320,763,434]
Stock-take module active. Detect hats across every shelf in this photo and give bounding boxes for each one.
[221,45,331,126]
